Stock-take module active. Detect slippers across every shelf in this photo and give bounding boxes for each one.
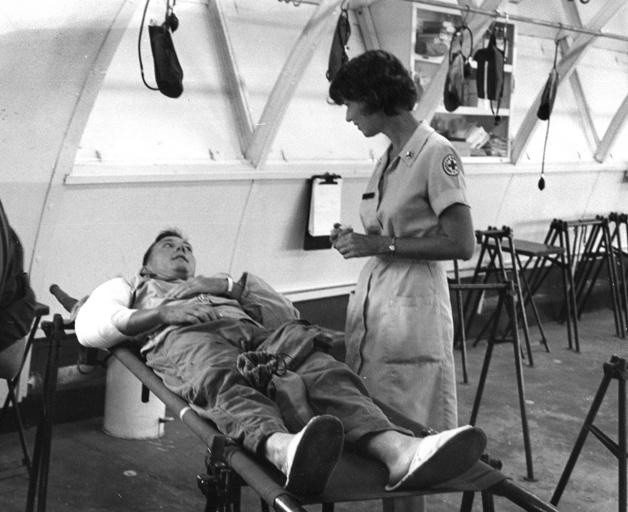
[283,415,345,493]
[385,425,486,492]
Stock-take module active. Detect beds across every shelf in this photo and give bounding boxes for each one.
[49,270,560,512]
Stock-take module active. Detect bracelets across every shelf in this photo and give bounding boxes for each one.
[223,277,234,297]
[389,236,396,259]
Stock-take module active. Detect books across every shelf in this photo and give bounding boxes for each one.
[415,20,508,158]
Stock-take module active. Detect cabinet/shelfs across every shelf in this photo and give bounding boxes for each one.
[407,1,517,164]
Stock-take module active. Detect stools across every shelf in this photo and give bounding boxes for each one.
[481,236,566,355]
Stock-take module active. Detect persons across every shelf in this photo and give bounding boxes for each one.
[325,49,476,510]
[75,229,487,500]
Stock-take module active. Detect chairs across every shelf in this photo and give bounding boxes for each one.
[0,298,51,476]
